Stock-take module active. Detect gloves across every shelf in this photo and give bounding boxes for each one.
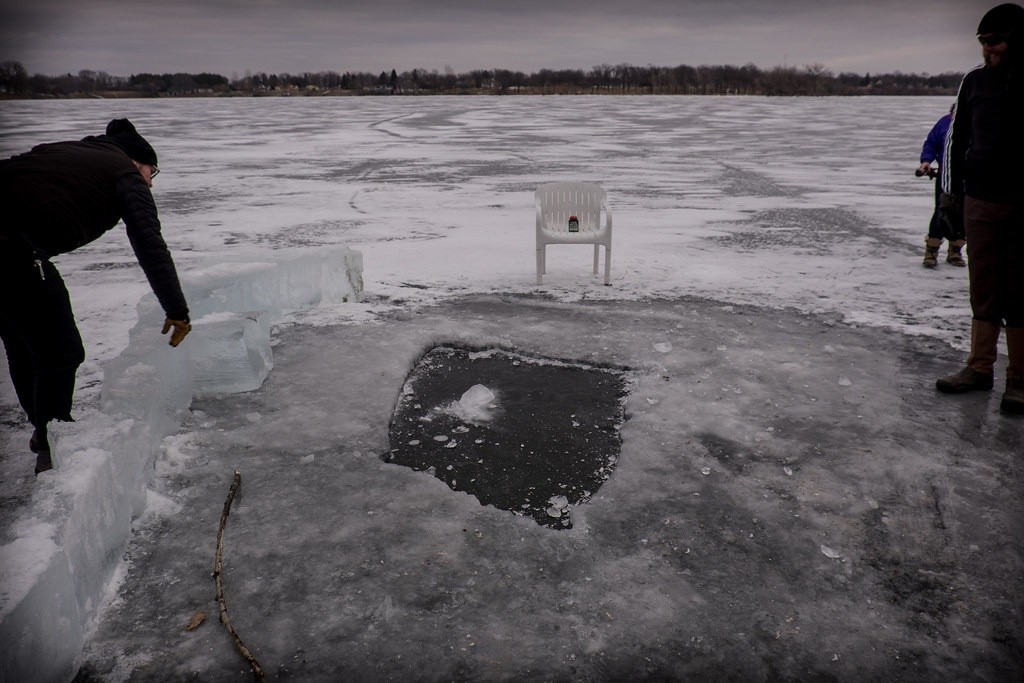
[161,314,192,347]
[939,194,960,222]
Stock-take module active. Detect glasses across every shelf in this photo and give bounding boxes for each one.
[978,34,1006,47]
[150,164,160,179]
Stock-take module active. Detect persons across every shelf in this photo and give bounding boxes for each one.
[917,105,966,267]
[936,3,1024,416]
[0,118,192,476]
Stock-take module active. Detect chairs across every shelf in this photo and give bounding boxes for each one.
[534,181,612,287]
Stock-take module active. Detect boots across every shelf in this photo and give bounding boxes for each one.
[945,239,967,267]
[935,317,1001,394]
[999,324,1024,417]
[923,236,943,267]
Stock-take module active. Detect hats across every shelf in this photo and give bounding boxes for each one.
[106,118,158,167]
[976,3,1024,35]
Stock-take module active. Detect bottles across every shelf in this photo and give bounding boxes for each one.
[916,167,927,176]
[569,216,579,232]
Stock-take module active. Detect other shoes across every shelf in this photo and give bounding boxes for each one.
[29,430,51,473]
